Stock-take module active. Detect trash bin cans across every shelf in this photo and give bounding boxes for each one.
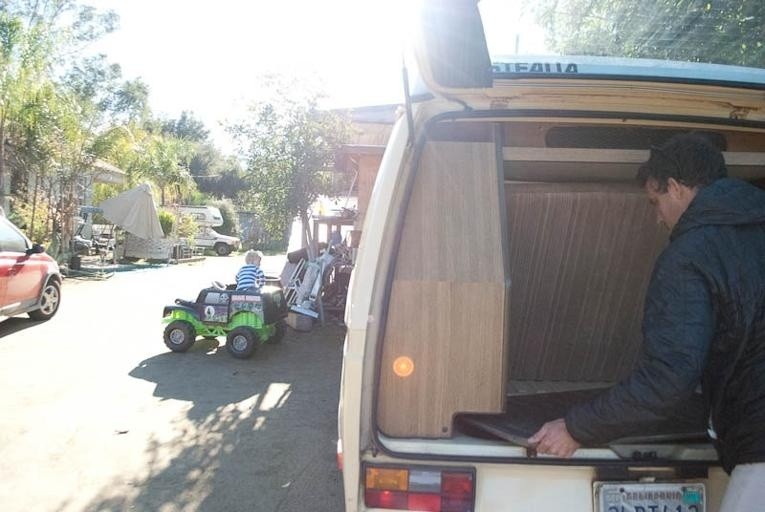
[71,257,81,269]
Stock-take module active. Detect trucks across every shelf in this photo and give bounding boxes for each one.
[166,203,244,257]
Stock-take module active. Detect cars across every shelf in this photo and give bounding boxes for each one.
[0,207,63,324]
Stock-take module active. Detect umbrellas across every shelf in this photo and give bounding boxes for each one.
[95,181,165,243]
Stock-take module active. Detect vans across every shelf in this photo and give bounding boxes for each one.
[337,0,765,510]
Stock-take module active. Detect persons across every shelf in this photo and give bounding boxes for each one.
[233,248,265,295]
[527,129,765,511]
